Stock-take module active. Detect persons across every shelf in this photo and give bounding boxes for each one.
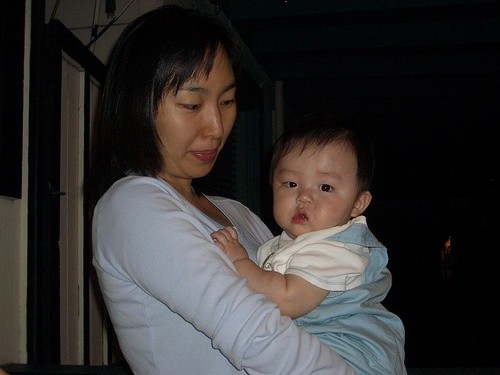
[209,116,407,375]
[91,5,360,375]
